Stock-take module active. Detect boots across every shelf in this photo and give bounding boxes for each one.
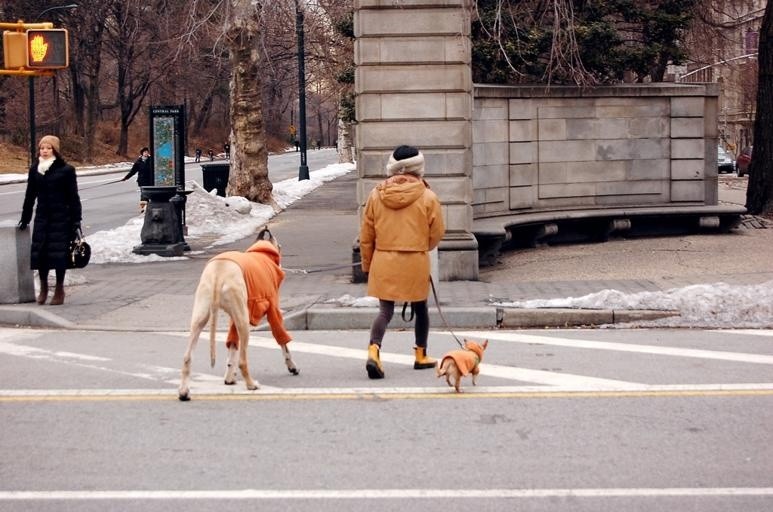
[414,346,437,368]
[366,345,384,378]
[37,289,47,304]
[139,201,146,212]
[52,286,64,304]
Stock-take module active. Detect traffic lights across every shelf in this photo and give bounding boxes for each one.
[24,29,69,71]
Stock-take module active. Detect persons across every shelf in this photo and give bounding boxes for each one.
[358,142,445,378]
[194,146,202,163]
[294,138,338,152]
[122,146,152,213]
[208,148,214,162]
[223,142,230,160]
[18,134,82,305]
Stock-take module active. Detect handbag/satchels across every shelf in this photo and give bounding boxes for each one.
[66,239,91,268]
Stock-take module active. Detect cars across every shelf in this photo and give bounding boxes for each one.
[734,146,753,177]
[717,145,733,174]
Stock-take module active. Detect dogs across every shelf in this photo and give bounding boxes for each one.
[435,339,488,392]
[177,227,299,403]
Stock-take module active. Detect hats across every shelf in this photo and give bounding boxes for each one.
[39,135,60,152]
[140,147,148,154]
